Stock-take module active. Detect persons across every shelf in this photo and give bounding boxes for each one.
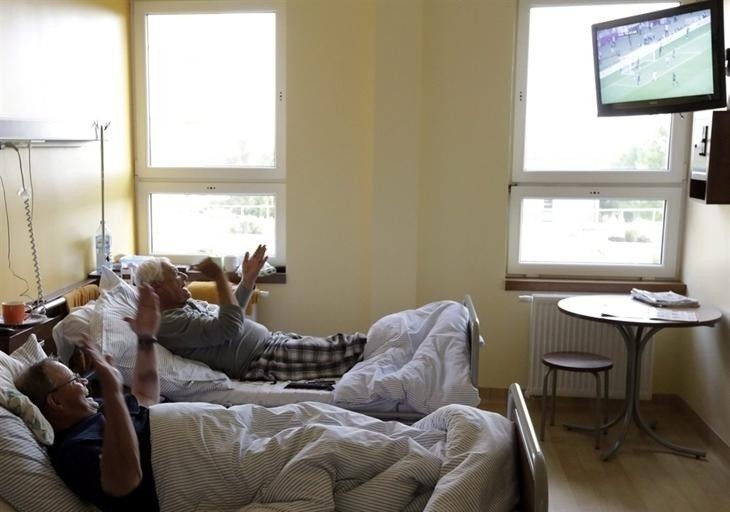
[12,283,162,511]
[133,244,368,383]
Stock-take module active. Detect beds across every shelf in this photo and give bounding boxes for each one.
[0,332,551,512]
[56,264,483,419]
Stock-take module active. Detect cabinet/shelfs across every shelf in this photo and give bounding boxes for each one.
[687,108,730,206]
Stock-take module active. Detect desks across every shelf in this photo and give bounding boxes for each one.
[555,295,727,464]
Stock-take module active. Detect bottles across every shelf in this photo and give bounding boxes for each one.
[95,219,112,274]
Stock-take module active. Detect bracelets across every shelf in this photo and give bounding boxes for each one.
[137,337,158,345]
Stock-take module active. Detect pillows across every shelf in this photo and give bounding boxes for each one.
[63,267,230,399]
[0,350,56,446]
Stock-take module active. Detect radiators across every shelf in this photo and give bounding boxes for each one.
[517,292,658,402]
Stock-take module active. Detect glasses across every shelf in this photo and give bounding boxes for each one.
[48,373,82,394]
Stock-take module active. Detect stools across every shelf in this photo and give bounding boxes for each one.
[537,351,614,451]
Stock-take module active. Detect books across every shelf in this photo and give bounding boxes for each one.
[630,288,700,308]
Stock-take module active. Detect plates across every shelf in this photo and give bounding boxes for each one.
[0,313,47,325]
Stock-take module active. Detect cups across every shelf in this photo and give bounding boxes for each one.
[2,301,32,324]
[224,255,243,272]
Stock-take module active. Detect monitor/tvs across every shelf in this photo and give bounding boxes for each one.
[591,0,727,118]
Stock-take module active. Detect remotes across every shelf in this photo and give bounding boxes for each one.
[284,381,335,390]
